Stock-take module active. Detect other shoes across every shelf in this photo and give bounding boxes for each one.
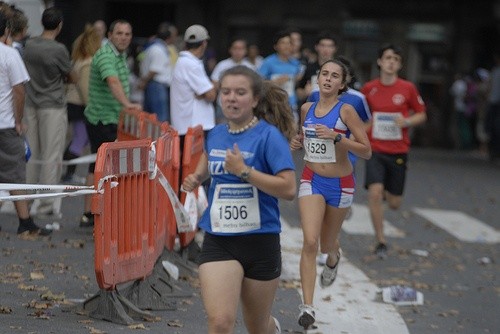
[64,175,86,185]
[272,317,281,334]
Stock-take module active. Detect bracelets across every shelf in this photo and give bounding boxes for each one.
[241,165,253,182]
[405,117,412,128]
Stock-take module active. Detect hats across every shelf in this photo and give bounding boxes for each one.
[184,25,210,43]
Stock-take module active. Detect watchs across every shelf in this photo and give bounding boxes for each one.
[334,133,343,145]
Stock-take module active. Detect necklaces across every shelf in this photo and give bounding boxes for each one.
[225,115,258,135]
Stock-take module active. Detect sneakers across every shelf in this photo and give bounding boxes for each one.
[79,215,94,227]
[374,243,389,260]
[298,304,315,330]
[17,222,54,241]
[320,247,343,289]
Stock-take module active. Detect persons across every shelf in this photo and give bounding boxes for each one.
[182,65,297,334]
[0,1,360,268]
[446,67,500,166]
[362,42,427,252]
[291,61,373,328]
